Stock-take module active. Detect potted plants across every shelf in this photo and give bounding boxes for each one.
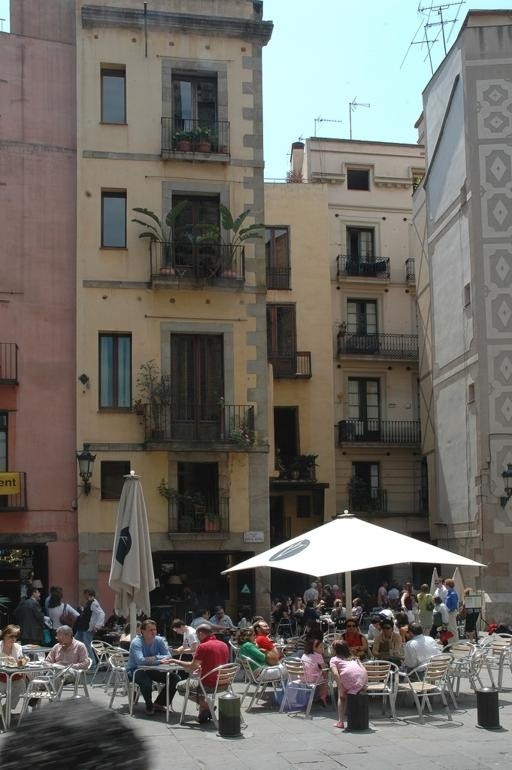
[204,511,223,533]
[171,128,192,152]
[135,358,176,441]
[130,200,196,277]
[288,462,302,479]
[134,399,145,416]
[194,125,213,153]
[157,478,195,534]
[196,204,267,280]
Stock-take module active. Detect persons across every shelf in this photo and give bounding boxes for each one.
[0,624,30,714]
[464,587,479,639]
[28,625,90,707]
[330,640,368,727]
[0,699,149,770]
[14,585,147,669]
[271,580,459,696]
[125,618,182,715]
[176,624,229,722]
[404,621,441,706]
[300,638,328,707]
[169,605,303,706]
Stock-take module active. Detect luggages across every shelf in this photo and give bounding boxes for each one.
[481,617,508,635]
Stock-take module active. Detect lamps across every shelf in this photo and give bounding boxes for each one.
[75,442,97,494]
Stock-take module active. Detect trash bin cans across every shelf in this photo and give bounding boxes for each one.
[152,606,174,643]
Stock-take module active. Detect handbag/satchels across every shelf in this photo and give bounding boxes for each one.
[440,629,453,642]
[60,604,75,625]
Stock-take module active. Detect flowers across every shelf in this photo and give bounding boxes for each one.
[227,416,257,450]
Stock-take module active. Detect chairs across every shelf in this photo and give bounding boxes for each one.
[0,594,512,732]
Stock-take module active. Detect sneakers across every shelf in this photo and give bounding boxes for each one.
[145,702,172,715]
[199,705,216,723]
[334,722,344,727]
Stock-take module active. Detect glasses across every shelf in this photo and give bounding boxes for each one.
[7,635,17,638]
[382,626,391,630]
[434,582,439,585]
[54,636,63,640]
[347,624,356,628]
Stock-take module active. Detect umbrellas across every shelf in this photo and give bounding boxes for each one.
[108,469,156,642]
[451,567,466,613]
[220,509,488,619]
[429,567,439,594]
